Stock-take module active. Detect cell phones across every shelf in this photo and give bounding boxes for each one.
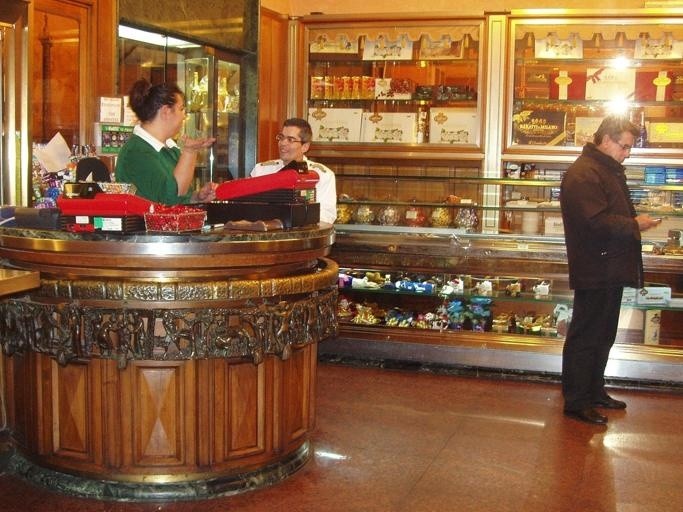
[650,215,667,222]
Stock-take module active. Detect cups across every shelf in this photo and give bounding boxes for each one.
[194,149,212,193]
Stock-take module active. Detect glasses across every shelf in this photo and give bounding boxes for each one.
[276,134,302,144]
[615,140,632,151]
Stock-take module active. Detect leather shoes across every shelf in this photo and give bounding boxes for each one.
[563,405,608,425]
[591,390,626,409]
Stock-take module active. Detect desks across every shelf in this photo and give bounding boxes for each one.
[0,222,339,509]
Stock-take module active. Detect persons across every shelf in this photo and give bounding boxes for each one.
[560,115,661,426]
[115,78,219,207]
[248,117,337,224]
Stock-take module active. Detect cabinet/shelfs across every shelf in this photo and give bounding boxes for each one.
[312,163,683,384]
